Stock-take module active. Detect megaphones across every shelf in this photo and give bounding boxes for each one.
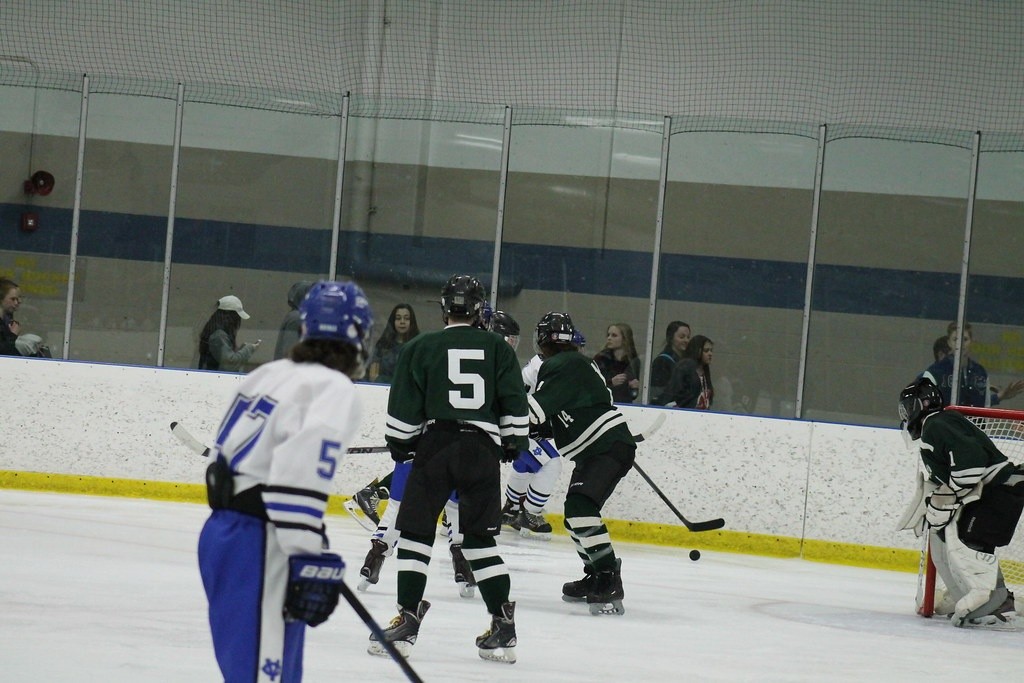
[29,170,55,197]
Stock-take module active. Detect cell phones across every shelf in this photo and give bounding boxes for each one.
[254,339,261,345]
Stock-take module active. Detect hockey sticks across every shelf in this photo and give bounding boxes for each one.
[632,461,726,532]
[529,410,669,443]
[915,521,929,609]
[337,580,423,683]
[170,420,390,457]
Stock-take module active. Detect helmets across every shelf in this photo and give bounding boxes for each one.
[533,311,574,354]
[482,300,493,331]
[492,310,520,352]
[440,274,486,325]
[299,279,373,367]
[898,378,944,441]
[288,282,311,309]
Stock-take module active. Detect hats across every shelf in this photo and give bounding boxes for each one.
[218,296,250,320]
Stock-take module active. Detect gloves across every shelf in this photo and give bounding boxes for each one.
[501,442,520,463]
[282,552,346,627]
[387,442,414,464]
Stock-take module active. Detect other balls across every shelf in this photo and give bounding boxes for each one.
[690,550,701,561]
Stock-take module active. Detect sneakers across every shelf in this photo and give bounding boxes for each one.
[476,600,517,664]
[367,600,431,659]
[357,538,388,591]
[501,498,521,533]
[449,543,478,597]
[518,496,552,540]
[440,511,448,536]
[562,557,625,615]
[344,486,388,532]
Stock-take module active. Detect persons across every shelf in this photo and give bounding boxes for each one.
[593,323,641,404]
[914,321,1024,408]
[274,281,312,362]
[0,278,52,358]
[198,295,262,372]
[649,321,691,405]
[500,330,587,533]
[342,310,526,527]
[360,300,495,587]
[527,310,637,604]
[369,273,517,649]
[364,303,421,383]
[659,335,714,410]
[198,282,375,683]
[898,378,1024,628]
